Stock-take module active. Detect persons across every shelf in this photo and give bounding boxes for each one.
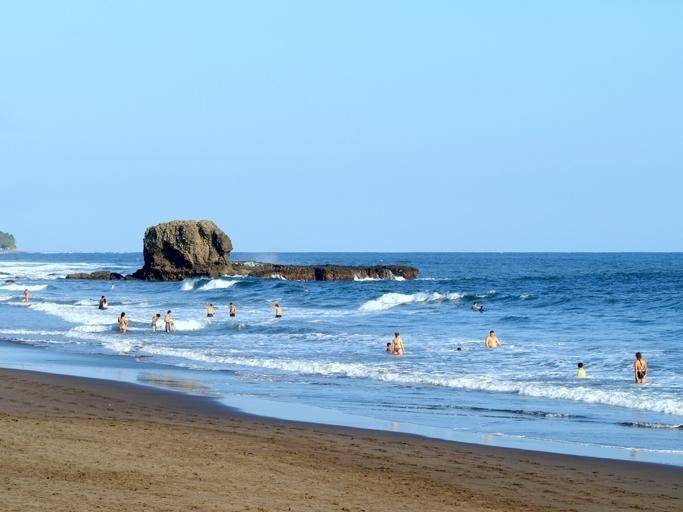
[169,321,175,332]
[386,342,393,354]
[478,305,484,311]
[23,288,28,302]
[98,295,105,309]
[634,352,647,384]
[151,312,160,332]
[116,311,127,334]
[391,330,403,355]
[110,282,114,288]
[471,301,478,310]
[456,347,462,351]
[274,303,282,318]
[228,303,236,317]
[163,309,172,332]
[576,362,585,377]
[484,329,501,349]
[206,303,215,318]
[100,299,107,309]
